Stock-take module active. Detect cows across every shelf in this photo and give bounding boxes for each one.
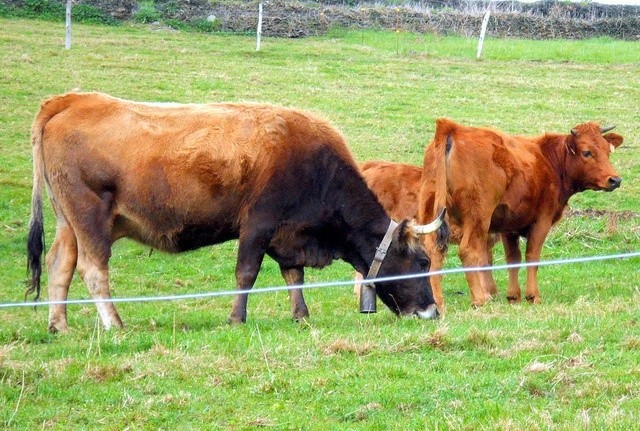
[352,159,501,303]
[20,91,447,336]
[417,118,623,316]
